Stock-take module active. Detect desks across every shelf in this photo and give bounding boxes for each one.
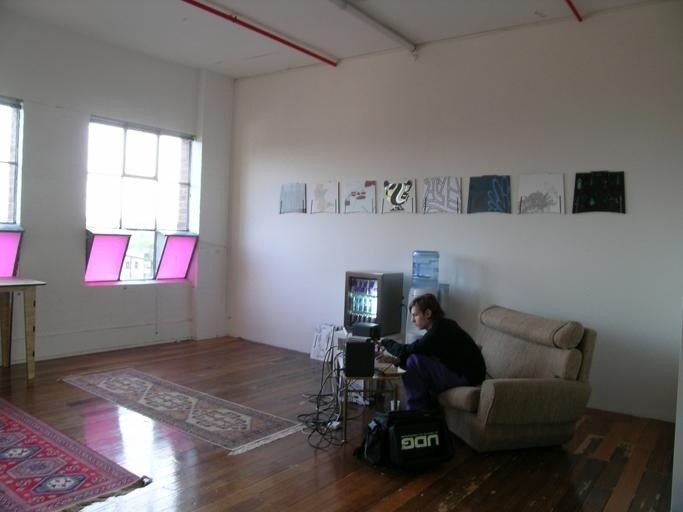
[0,277,48,383]
[336,351,405,445]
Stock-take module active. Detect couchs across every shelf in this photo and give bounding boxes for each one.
[440,305,598,456]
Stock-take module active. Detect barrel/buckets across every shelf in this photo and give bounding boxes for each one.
[411,249,441,289]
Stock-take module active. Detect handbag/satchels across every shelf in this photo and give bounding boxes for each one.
[361,407,454,465]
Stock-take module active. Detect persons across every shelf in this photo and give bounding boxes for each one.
[372,293,485,412]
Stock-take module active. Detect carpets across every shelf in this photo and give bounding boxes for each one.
[59,365,302,455]
[1,398,151,509]
